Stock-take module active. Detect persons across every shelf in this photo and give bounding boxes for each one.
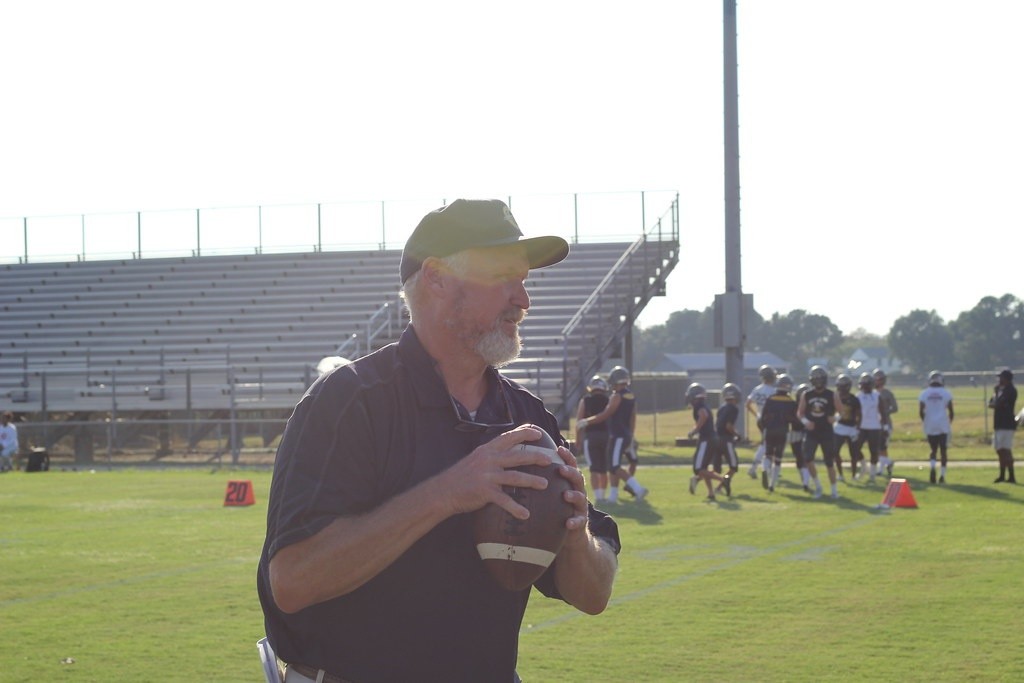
[575,365,648,505]
[855,371,887,482]
[832,374,862,481]
[0,411,19,471]
[577,375,611,502]
[791,383,810,492]
[796,365,844,499]
[686,382,731,500]
[746,365,778,478]
[920,370,953,483]
[762,373,796,492]
[689,382,742,496]
[871,369,897,475]
[988,370,1018,483]
[256,200,620,683]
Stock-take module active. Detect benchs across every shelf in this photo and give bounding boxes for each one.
[0,240,679,404]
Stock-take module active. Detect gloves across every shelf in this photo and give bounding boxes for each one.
[732,431,742,443]
[576,419,588,429]
[688,432,696,439]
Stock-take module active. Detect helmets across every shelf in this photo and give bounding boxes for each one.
[797,383,809,395]
[589,375,606,392]
[606,367,630,385]
[808,366,826,384]
[874,371,886,380]
[758,364,777,380]
[722,383,741,400]
[836,374,852,384]
[776,374,793,392]
[687,383,706,398]
[928,370,943,385]
[857,372,874,383]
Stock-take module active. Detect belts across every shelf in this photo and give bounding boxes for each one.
[288,662,353,683]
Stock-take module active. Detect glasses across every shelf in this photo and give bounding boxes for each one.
[436,364,514,435]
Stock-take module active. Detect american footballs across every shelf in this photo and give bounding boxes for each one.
[471,423,577,596]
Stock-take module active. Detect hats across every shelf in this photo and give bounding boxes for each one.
[996,370,1013,379]
[399,200,569,287]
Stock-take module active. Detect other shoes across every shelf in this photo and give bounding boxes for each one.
[592,485,648,507]
[689,460,1015,503]
